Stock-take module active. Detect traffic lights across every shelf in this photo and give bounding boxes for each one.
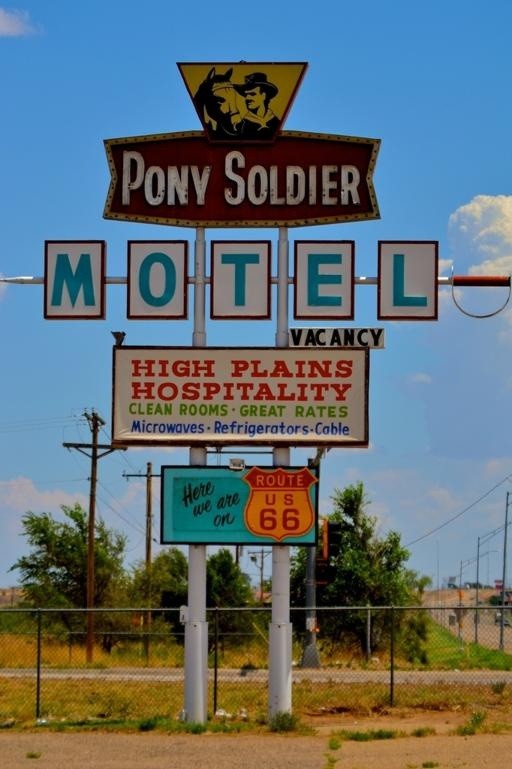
[316,559,337,586]
[323,519,343,560]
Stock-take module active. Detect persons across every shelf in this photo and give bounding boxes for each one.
[232,72,279,137]
